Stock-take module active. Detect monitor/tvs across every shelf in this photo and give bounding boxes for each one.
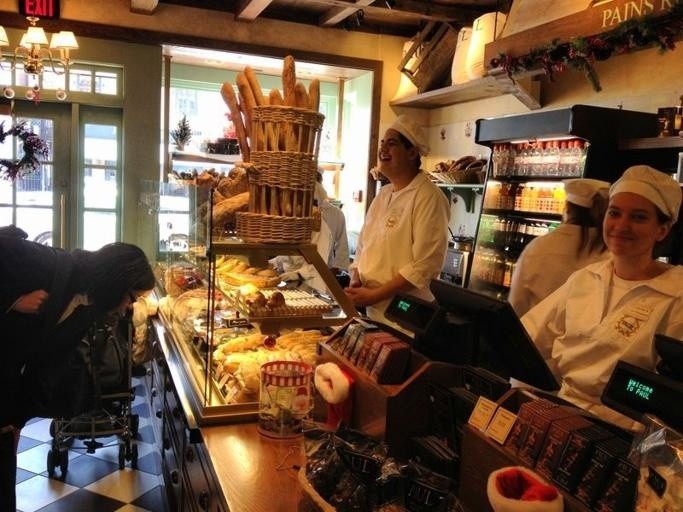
[429,278,561,391]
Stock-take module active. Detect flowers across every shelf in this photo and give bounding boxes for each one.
[0,118,50,183]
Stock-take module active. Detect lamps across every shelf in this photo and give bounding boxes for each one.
[0,16,78,104]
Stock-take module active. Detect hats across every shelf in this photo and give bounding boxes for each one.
[608,165,682,224]
[386,114,431,158]
[562,177,610,209]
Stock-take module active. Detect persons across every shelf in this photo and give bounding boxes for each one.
[521,166,683,399]
[345,115,450,336]
[310,167,349,278]
[0,225,156,512]
[506,177,610,319]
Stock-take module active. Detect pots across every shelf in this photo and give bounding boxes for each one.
[451,236,474,252]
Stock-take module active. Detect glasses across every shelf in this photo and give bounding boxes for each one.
[127,291,137,305]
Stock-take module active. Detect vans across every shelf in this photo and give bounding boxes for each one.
[0,138,236,251]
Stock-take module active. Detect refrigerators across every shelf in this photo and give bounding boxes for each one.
[463,104,662,293]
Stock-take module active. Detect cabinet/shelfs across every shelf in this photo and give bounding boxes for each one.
[627,135,682,263]
[464,104,658,304]
[390,76,540,290]
[143,319,228,512]
[155,243,361,426]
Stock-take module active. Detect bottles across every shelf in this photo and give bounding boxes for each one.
[566,142,575,175]
[493,218,562,255]
[534,142,543,175]
[483,180,513,209]
[477,214,498,242]
[543,143,552,175]
[582,142,589,176]
[560,143,566,176]
[548,142,561,176]
[470,243,519,288]
[522,182,567,214]
[515,183,525,210]
[570,141,581,177]
[493,142,535,177]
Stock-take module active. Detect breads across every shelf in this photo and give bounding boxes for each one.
[159,263,204,317]
[214,254,282,291]
[267,292,285,310]
[174,289,250,358]
[212,329,332,403]
[246,291,264,311]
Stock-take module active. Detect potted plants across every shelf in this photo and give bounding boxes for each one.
[170,113,193,151]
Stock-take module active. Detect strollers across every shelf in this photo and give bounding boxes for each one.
[48,305,138,474]
